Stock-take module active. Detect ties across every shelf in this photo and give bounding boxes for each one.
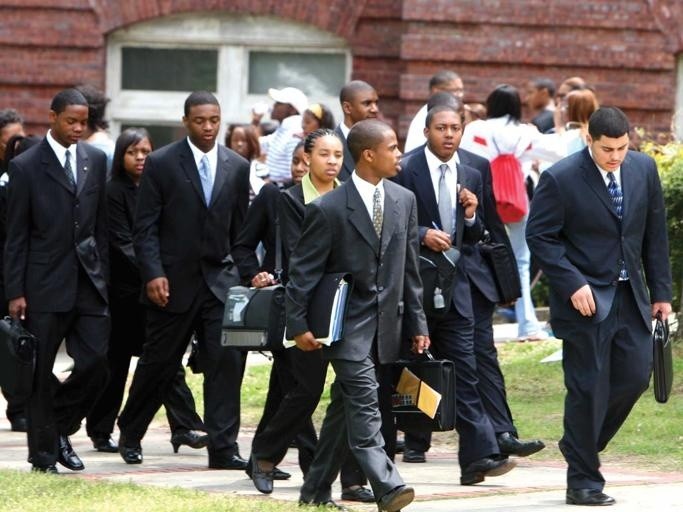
[372,187,383,240]
[197,155,212,207]
[63,150,75,189]
[438,164,452,244]
[608,172,627,280]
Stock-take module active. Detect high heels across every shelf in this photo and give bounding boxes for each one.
[171,430,208,453]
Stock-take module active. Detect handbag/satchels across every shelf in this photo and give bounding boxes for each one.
[379,348,457,431]
[220,283,287,352]
[418,245,461,318]
[478,239,522,302]
[1,317,37,406]
[653,311,672,403]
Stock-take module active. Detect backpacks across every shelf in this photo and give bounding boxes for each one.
[484,120,537,224]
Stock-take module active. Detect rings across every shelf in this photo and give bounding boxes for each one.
[260,278,267,283]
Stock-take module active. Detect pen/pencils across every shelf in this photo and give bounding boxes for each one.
[431,221,442,232]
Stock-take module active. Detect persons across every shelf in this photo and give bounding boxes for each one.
[388,91,546,486]
[524,106,674,506]
[0,88,158,474]
[224,79,390,267]
[402,73,598,343]
[231,128,376,504]
[116,91,252,469]
[283,120,414,512]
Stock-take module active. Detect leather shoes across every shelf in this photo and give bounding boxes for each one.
[298,484,414,512]
[497,433,546,459]
[10,415,142,474]
[461,457,517,484]
[404,445,425,462]
[559,435,616,505]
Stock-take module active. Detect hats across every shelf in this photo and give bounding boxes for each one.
[268,87,308,114]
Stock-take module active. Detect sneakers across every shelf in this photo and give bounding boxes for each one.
[210,445,290,493]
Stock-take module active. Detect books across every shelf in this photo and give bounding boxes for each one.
[285,274,352,349]
